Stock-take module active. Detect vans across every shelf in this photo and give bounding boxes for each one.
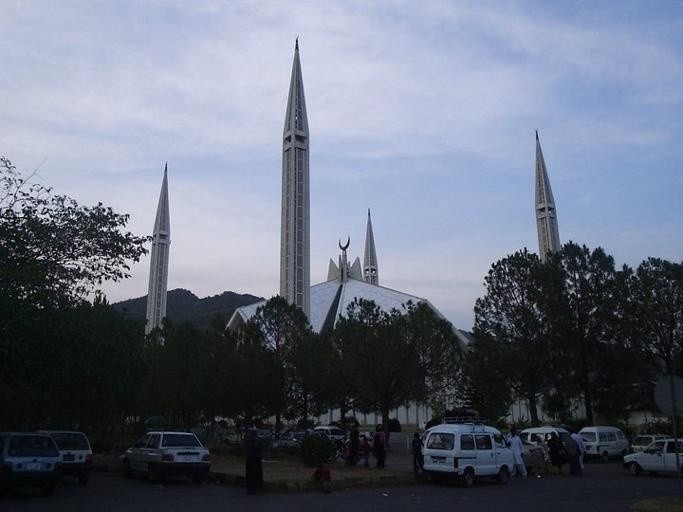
[313,425,346,441]
[631,433,672,453]
[518,420,630,462]
[2,428,95,498]
[419,423,514,488]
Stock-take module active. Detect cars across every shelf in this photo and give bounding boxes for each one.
[623,438,683,476]
[122,429,212,482]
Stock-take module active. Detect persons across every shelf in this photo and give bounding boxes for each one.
[345,423,390,469]
[548,431,588,475]
[411,433,424,478]
[645,443,664,453]
[242,430,265,496]
[506,428,528,481]
[313,458,335,493]
